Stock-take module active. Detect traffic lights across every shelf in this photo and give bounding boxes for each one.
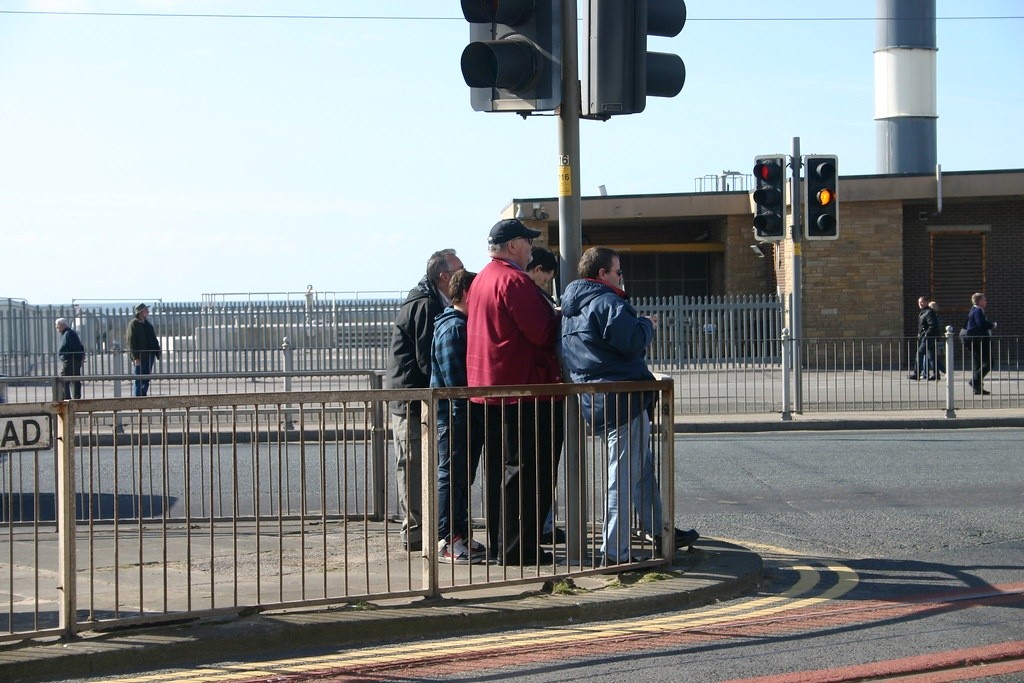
[753,153,786,243]
[802,154,839,241]
[460,2,562,114]
[584,1,686,119]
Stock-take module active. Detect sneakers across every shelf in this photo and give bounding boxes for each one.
[438,532,482,564]
[599,555,650,575]
[461,531,486,556]
[655,528,699,556]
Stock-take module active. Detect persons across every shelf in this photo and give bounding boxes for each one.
[907,296,946,381]
[524,246,566,545]
[382,248,464,551]
[55,317,87,400]
[426,268,487,565]
[561,245,700,575]
[463,217,555,566]
[126,303,161,397]
[966,292,997,395]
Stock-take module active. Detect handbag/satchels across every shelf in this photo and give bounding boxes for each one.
[959,328,970,348]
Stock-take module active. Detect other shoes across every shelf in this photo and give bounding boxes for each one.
[969,379,982,390]
[975,388,990,395]
[929,374,941,380]
[907,374,920,380]
[540,527,565,544]
[497,547,553,566]
[403,542,422,551]
[921,375,925,380]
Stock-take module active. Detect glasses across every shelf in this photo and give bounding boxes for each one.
[511,237,533,244]
[443,267,463,273]
[605,269,622,276]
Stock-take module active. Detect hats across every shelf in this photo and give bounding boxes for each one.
[488,219,542,244]
[133,303,151,317]
